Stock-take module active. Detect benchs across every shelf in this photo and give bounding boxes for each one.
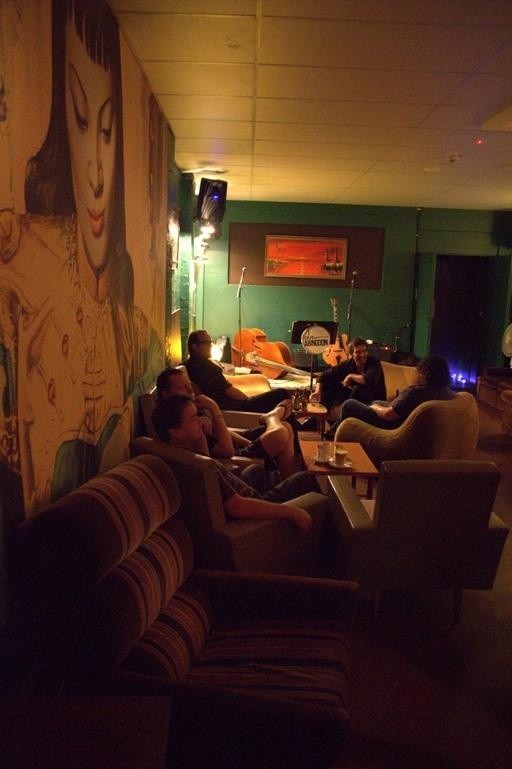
[477,366,512,415]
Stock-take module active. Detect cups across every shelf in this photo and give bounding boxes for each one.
[334,449,347,465]
[317,442,331,460]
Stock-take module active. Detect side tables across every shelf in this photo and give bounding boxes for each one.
[0,692,172,769]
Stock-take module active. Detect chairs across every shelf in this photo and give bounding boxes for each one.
[129,436,332,613]
[500,390,512,439]
[13,454,363,768]
[379,360,422,402]
[327,457,509,624]
[333,390,480,485]
[174,364,272,429]
[141,383,247,440]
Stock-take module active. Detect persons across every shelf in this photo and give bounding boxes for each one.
[156,368,297,479]
[307,336,387,441]
[1,0,166,527]
[151,393,328,535]
[182,330,303,454]
[321,354,457,441]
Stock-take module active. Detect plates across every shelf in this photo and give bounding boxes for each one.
[311,455,333,464]
[329,461,352,469]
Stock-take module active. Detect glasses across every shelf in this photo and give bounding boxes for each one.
[192,340,213,344]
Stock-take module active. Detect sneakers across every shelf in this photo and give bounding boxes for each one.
[325,421,338,440]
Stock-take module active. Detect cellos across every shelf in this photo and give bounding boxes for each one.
[231,328,323,380]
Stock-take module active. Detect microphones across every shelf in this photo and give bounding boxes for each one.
[241,266,247,276]
[351,270,358,283]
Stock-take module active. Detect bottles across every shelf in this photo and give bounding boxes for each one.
[294,388,308,411]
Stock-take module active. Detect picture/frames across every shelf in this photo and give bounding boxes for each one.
[263,234,348,280]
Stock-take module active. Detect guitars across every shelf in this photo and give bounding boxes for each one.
[322,297,352,368]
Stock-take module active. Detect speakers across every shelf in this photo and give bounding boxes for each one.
[197,177,228,225]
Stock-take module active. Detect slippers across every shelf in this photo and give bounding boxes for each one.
[273,399,294,421]
